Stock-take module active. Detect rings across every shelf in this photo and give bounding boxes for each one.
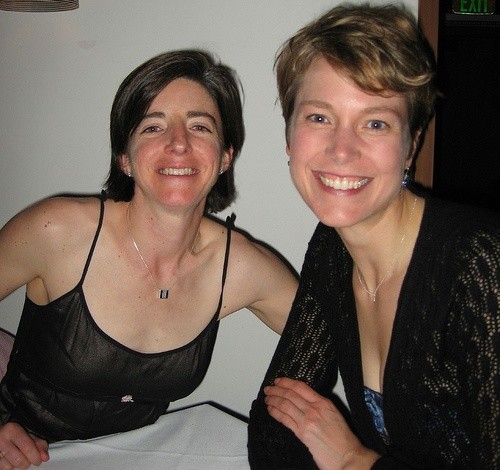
[0,452,3,459]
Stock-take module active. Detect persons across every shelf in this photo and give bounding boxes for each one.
[247,2,500,470]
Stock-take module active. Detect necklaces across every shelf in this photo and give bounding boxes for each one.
[0,49,300,470]
[355,194,418,303]
[126,199,204,300]
